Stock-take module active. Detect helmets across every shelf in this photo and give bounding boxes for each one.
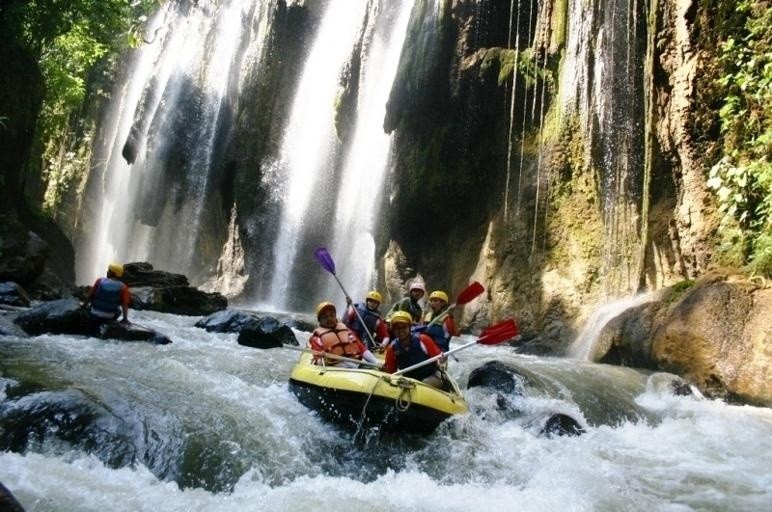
[430,290,449,305]
[317,302,336,320]
[390,312,412,325]
[108,264,123,277]
[409,283,424,296]
[366,291,382,303]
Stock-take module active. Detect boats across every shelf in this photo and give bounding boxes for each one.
[288,335,469,440]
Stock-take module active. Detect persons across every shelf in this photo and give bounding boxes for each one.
[420,290,461,352]
[309,301,385,371]
[341,291,391,353]
[384,282,425,326]
[386,310,447,390]
[80,262,131,325]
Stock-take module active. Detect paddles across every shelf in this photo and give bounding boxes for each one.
[392,319,519,375]
[424,282,484,334]
[315,248,378,347]
